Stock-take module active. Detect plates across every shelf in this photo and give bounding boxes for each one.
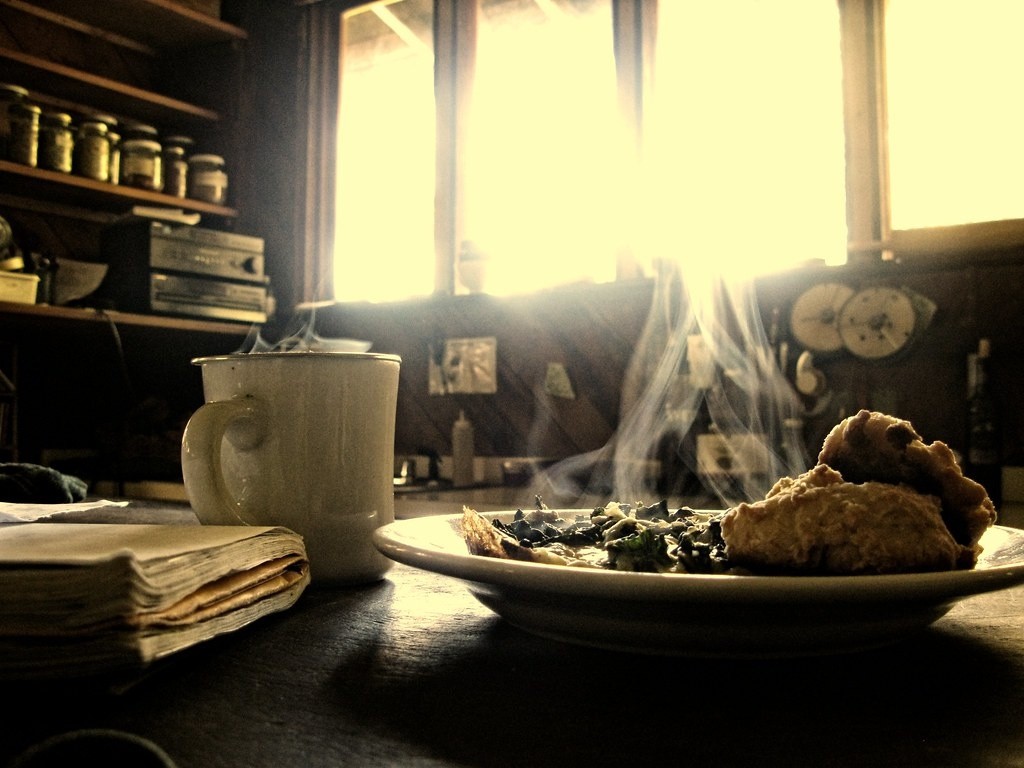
[372,507,1024,637]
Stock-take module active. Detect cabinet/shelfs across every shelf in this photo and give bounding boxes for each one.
[1,0,275,489]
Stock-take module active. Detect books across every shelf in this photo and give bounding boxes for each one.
[0,521,313,669]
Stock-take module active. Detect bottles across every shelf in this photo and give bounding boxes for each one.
[779,420,813,478]
[959,355,997,494]
[453,409,474,488]
[13,103,229,204]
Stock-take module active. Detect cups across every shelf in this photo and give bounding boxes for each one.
[410,455,429,477]
[181,352,402,581]
[437,456,551,484]
[1001,467,1024,506]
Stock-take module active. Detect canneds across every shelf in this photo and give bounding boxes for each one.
[6,101,231,205]
